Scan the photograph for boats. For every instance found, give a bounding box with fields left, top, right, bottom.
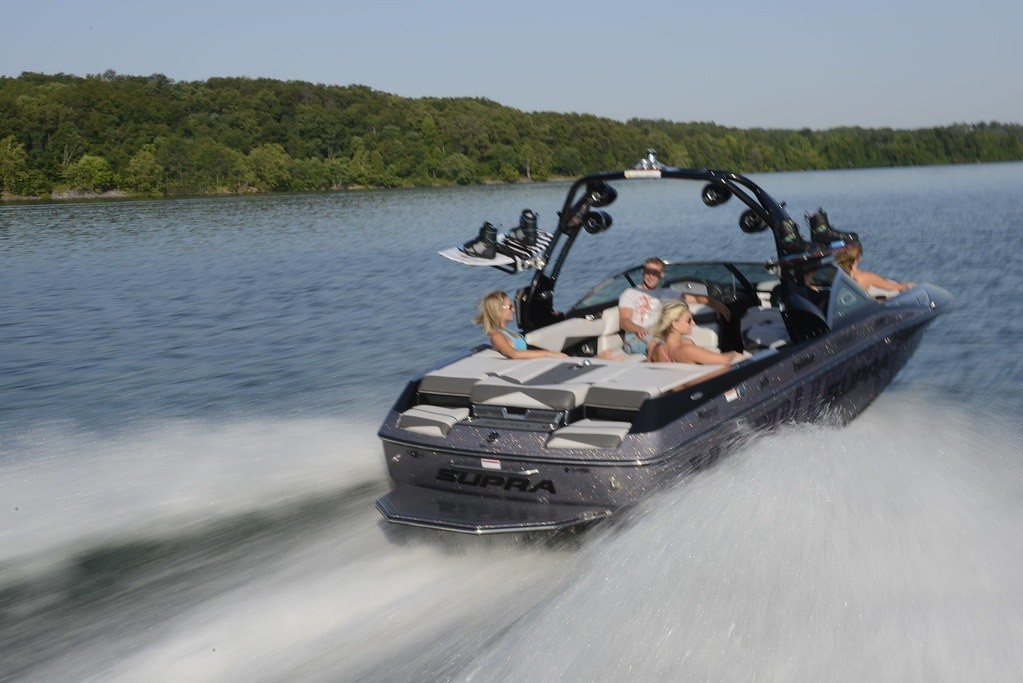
left=373, top=150, right=958, bottom=535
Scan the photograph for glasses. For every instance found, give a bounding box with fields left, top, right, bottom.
left=502, top=304, right=513, bottom=309
left=682, top=318, right=692, bottom=324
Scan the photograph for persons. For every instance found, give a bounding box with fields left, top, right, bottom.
left=470, top=290, right=627, bottom=360
left=839, top=243, right=918, bottom=298
left=646, top=299, right=745, bottom=365
left=618, top=255, right=731, bottom=359
